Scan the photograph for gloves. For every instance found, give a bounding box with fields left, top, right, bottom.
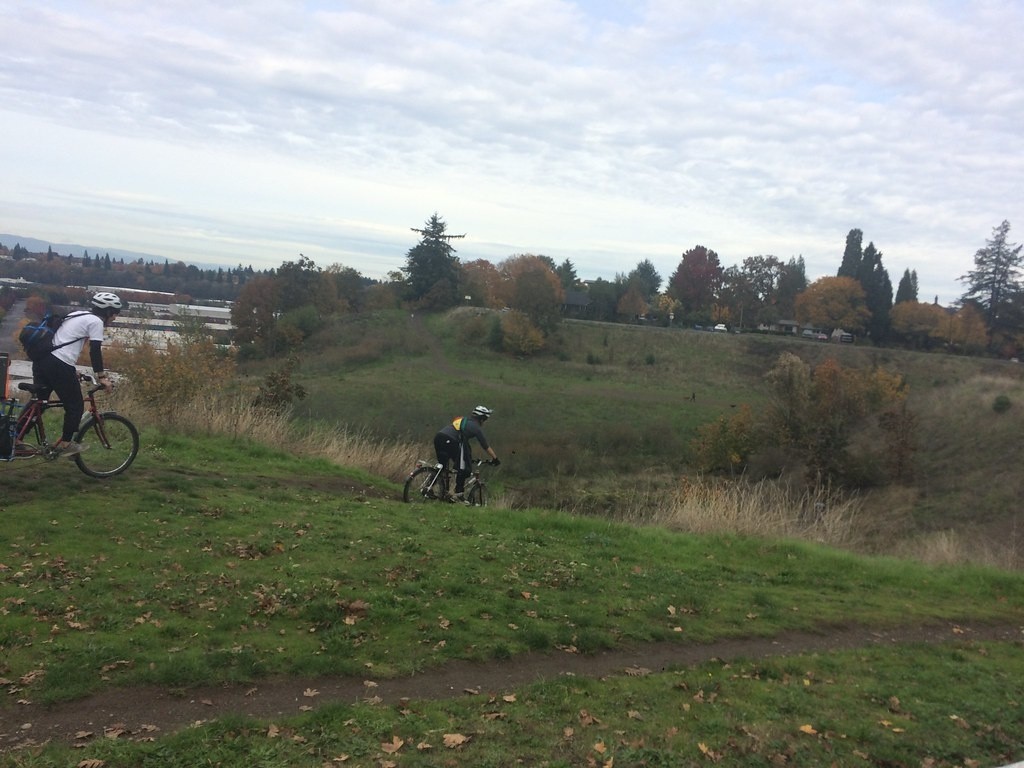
left=490, top=457, right=501, bottom=467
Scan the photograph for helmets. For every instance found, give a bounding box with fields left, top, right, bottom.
left=91, top=292, right=123, bottom=309
left=472, top=405, right=491, bottom=418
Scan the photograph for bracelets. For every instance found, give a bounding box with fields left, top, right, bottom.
left=98, top=375, right=106, bottom=379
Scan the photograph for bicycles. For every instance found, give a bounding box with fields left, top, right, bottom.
left=403, top=455, right=491, bottom=509
left=0, top=373, right=141, bottom=478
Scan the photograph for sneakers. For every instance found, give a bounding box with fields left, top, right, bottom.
left=0, top=446, right=35, bottom=462
left=58, top=440, right=89, bottom=457
left=451, top=494, right=470, bottom=506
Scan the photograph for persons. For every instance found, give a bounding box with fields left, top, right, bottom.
left=434, top=405, right=501, bottom=505
left=12, top=291, right=122, bottom=458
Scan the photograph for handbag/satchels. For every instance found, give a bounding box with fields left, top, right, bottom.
left=456, top=439, right=473, bottom=478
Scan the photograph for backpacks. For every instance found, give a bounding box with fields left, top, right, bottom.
left=19, top=308, right=98, bottom=362
